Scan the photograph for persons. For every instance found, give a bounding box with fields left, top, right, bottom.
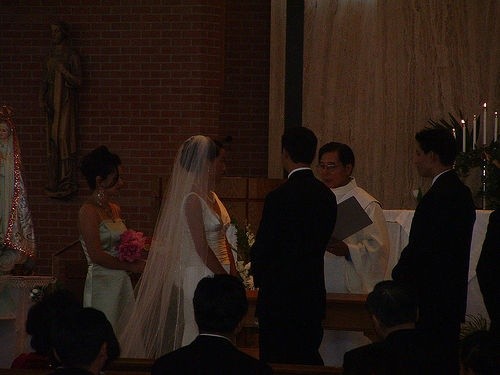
left=149, top=273, right=279, bottom=375
left=242, top=125, right=337, bottom=362
left=341, top=281, right=462, bottom=375
left=78, top=144, right=150, bottom=358
left=476, top=210, right=500, bottom=342
left=112, top=135, right=245, bottom=359
left=392, top=127, right=475, bottom=375
left=11, top=298, right=122, bottom=375
left=0, top=103, right=39, bottom=275
left=41, top=22, right=81, bottom=197
left=318, top=141, right=391, bottom=296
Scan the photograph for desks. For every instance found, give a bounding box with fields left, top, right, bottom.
left=323, top=209, right=496, bottom=339
left=0, top=275, right=57, bottom=370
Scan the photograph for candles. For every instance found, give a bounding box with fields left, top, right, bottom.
left=453, top=128, right=456, bottom=170
left=483, top=103, right=487, bottom=144
left=473, top=114, right=476, bottom=150
left=494, top=112, right=498, bottom=143
left=461, top=120, right=466, bottom=153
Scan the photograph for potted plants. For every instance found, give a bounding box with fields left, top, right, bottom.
left=424, top=108, right=500, bottom=197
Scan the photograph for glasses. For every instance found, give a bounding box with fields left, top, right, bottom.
left=315, top=164, right=342, bottom=171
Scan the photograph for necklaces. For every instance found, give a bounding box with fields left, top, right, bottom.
left=201, top=189, right=218, bottom=204
left=92, top=198, right=119, bottom=223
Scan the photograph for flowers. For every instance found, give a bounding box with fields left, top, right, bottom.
left=118, top=229, right=152, bottom=275
left=412, top=187, right=422, bottom=208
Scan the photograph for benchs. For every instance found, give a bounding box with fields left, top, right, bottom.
left=21, top=357, right=347, bottom=375
